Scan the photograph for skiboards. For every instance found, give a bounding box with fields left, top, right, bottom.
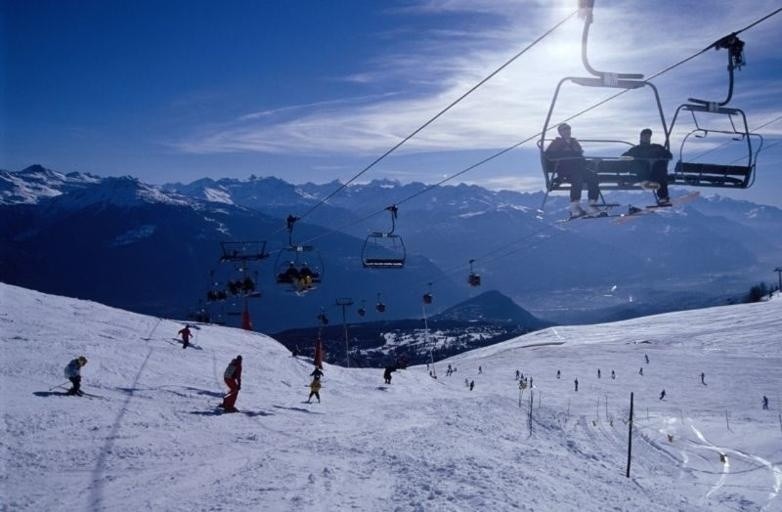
left=213, top=402, right=242, bottom=414
left=613, top=188, right=700, bottom=223
left=559, top=204, right=621, bottom=225
left=59, top=385, right=102, bottom=401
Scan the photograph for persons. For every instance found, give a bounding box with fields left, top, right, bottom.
left=64, top=355, right=88, bottom=397
left=310, top=366, right=323, bottom=381
left=206, top=276, right=255, bottom=303
left=177, top=323, right=194, bottom=350
left=426, top=350, right=706, bottom=402
left=307, top=375, right=322, bottom=403
left=620, top=128, right=674, bottom=205
left=284, top=261, right=300, bottom=287
left=298, top=261, right=315, bottom=285
left=542, top=123, right=609, bottom=218
left=761, top=395, right=770, bottom=411
left=383, top=362, right=396, bottom=384
left=221, top=355, right=243, bottom=413
